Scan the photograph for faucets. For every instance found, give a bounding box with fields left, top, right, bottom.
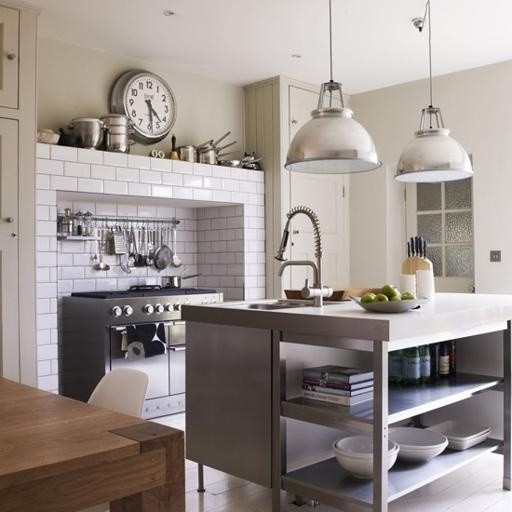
left=278, top=260, right=318, bottom=284
left=273, top=205, right=323, bottom=307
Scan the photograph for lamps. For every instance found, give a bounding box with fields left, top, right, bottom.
left=284, top=0, right=383, bottom=174
left=393, top=0, right=472, bottom=183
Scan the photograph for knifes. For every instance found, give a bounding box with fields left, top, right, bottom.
left=407, top=236, right=427, bottom=260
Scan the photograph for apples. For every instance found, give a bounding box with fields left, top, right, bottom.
left=358, top=283, right=415, bottom=303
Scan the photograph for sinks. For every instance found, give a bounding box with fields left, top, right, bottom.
left=272, top=299, right=332, bottom=307
left=247, top=304, right=286, bottom=310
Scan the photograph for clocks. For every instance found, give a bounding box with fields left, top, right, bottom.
left=108, top=68, right=177, bottom=145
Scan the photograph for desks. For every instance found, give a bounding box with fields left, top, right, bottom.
left=0, top=377, right=185, bottom=512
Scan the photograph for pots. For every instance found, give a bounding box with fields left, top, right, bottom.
left=68, top=118, right=108, bottom=150
left=97, top=113, right=136, bottom=152
left=160, top=272, right=202, bottom=289
left=179, top=130, right=265, bottom=171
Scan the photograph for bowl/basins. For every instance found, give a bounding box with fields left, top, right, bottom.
left=36, top=130, right=60, bottom=145
left=332, top=434, right=400, bottom=480
left=58, top=127, right=82, bottom=147
left=381, top=427, right=448, bottom=463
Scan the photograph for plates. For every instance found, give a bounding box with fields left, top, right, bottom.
left=349, top=295, right=427, bottom=312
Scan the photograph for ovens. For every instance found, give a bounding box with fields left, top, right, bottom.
left=62, top=293, right=225, bottom=420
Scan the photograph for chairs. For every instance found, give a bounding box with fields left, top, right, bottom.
left=87, top=367, right=149, bottom=418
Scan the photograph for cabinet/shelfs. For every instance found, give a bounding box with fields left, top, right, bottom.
left=288, top=84, right=347, bottom=290
left=281, top=371, right=503, bottom=503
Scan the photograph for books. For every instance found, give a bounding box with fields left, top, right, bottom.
left=301, top=365, right=374, bottom=407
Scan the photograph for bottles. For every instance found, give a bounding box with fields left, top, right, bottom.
left=389, top=339, right=456, bottom=386
left=60, top=208, right=94, bottom=235
left=171, top=150, right=179, bottom=161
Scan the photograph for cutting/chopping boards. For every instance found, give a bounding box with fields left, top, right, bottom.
left=284, top=288, right=384, bottom=301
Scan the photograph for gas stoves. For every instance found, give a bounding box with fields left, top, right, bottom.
left=62, top=284, right=223, bottom=324
